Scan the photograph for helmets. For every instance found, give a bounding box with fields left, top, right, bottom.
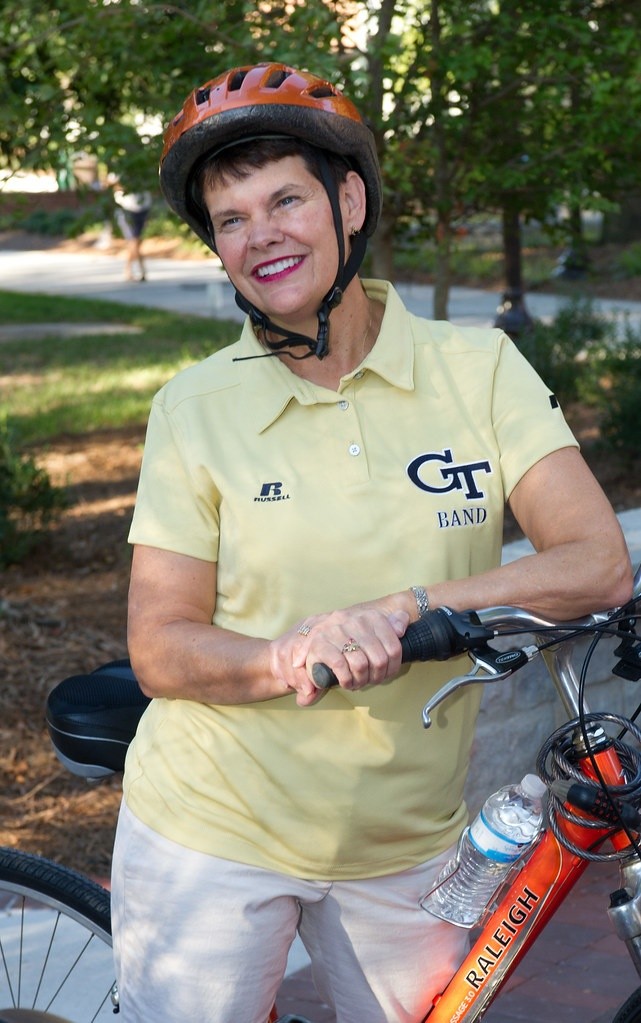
left=159, top=62, right=382, bottom=254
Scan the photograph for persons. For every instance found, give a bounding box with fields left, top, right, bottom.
left=105, top=61, right=639, bottom=1023
left=102, top=164, right=150, bottom=283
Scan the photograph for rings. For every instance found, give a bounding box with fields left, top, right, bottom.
left=297, top=625, right=311, bottom=636
left=342, top=638, right=360, bottom=655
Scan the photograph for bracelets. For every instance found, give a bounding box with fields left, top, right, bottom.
left=409, top=585, right=429, bottom=619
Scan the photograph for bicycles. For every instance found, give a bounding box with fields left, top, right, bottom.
left=2, top=596, right=641, bottom=1023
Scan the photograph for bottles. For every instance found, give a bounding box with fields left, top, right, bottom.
left=431, top=774, right=546, bottom=925
